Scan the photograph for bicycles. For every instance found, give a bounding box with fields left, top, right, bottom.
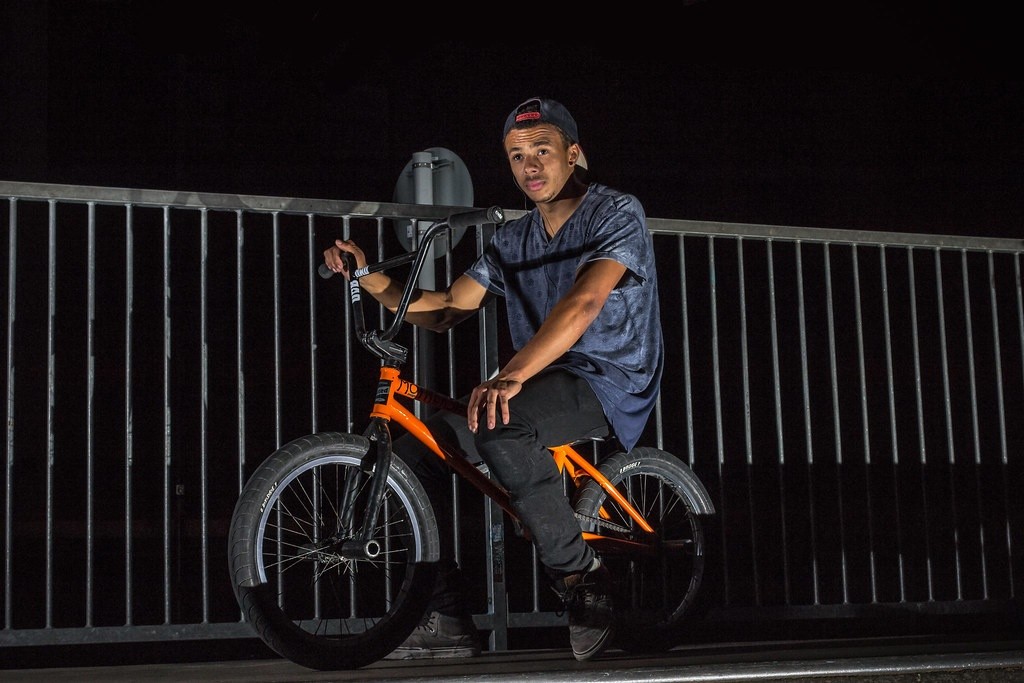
left=226, top=201, right=720, bottom=672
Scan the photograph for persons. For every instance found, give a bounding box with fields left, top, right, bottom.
left=321, top=94, right=669, bottom=667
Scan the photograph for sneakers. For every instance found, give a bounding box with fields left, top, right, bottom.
left=380, top=606, right=482, bottom=658
left=554, top=559, right=622, bottom=661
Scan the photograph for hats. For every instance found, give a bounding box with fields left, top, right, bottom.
left=501, top=97, right=588, bottom=170
